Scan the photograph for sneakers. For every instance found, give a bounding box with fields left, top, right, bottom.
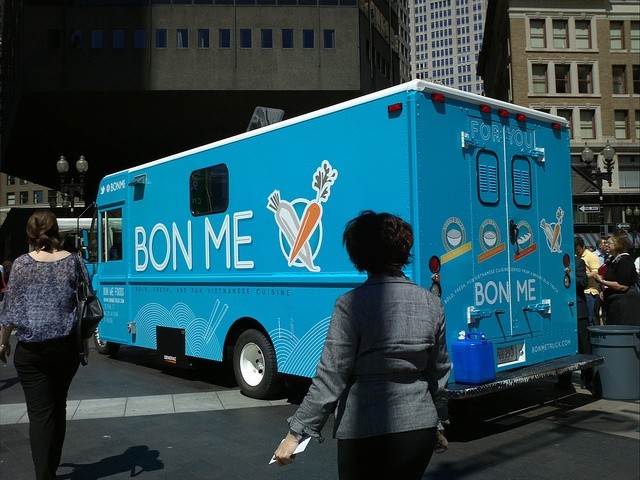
left=580, top=380, right=591, bottom=389
left=553, top=382, right=570, bottom=387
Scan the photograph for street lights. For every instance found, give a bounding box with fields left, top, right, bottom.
left=56, top=154, right=92, bottom=207
left=625, top=204, right=640, bottom=247
left=580, top=140, right=616, bottom=238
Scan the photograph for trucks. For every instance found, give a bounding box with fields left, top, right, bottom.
left=73, top=77, right=605, bottom=431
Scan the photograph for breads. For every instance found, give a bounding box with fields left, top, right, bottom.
left=517, top=233, right=531, bottom=244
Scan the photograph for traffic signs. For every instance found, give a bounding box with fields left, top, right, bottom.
left=578, top=204, right=600, bottom=213
left=616, top=223, right=630, bottom=229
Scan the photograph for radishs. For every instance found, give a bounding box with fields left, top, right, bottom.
left=266, top=189, right=321, bottom=272
left=540, top=219, right=562, bottom=253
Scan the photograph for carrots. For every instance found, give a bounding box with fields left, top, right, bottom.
left=551, top=206, right=564, bottom=250
left=289, top=160, right=337, bottom=264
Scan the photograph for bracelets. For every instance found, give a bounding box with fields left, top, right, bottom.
left=0, top=343, right=12, bottom=348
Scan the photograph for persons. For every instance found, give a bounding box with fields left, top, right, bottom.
left=589, top=245, right=596, bottom=253
left=589, top=239, right=608, bottom=269
left=576, top=236, right=605, bottom=339
left=603, top=235, right=616, bottom=267
left=542, top=239, right=593, bottom=396
left=1, top=259, right=12, bottom=302
left=272, top=209, right=452, bottom=480
left=0, top=207, right=95, bottom=479
left=593, top=233, right=640, bottom=326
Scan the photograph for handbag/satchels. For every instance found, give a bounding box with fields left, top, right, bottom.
left=77, top=280, right=104, bottom=338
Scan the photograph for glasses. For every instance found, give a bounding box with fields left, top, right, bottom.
left=604, top=242, right=617, bottom=246
left=575, top=247, right=581, bottom=253
